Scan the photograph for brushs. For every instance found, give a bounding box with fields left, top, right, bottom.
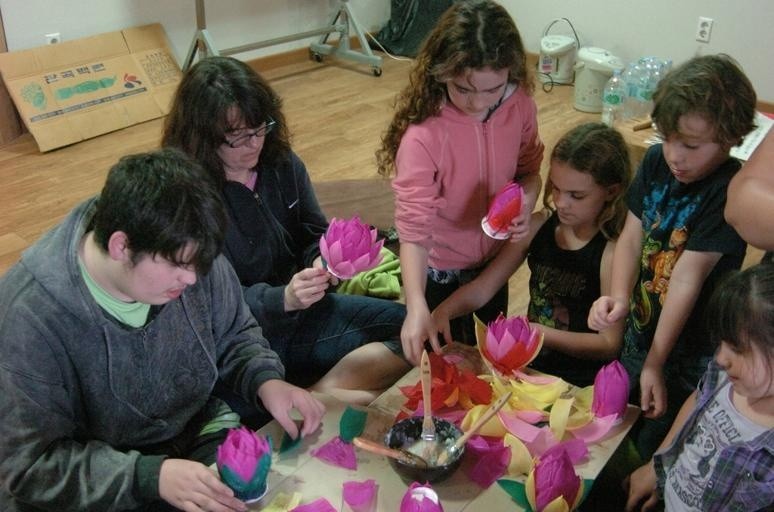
left=421, top=349, right=438, bottom=467
left=353, top=437, right=428, bottom=469
left=436, top=392, right=512, bottom=467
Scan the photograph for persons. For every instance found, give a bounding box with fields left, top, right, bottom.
left=0, top=147, right=328, bottom=512
left=619, top=259, right=773, bottom=512
left=587, top=52, right=757, bottom=463
left=722, top=124, right=774, bottom=264
left=430, top=120, right=633, bottom=389
left=374, top=0, right=545, bottom=365
left=162, top=55, right=414, bottom=405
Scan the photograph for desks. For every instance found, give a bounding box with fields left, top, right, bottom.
left=194, top=338, right=645, bottom=512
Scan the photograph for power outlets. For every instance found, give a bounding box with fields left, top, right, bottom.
left=44, top=33, right=60, bottom=46
left=694, top=17, right=713, bottom=42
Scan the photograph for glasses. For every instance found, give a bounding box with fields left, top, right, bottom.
left=223, top=115, right=276, bottom=147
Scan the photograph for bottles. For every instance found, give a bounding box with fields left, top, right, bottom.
left=601, top=67, right=629, bottom=134
left=625, top=55, right=675, bottom=117
